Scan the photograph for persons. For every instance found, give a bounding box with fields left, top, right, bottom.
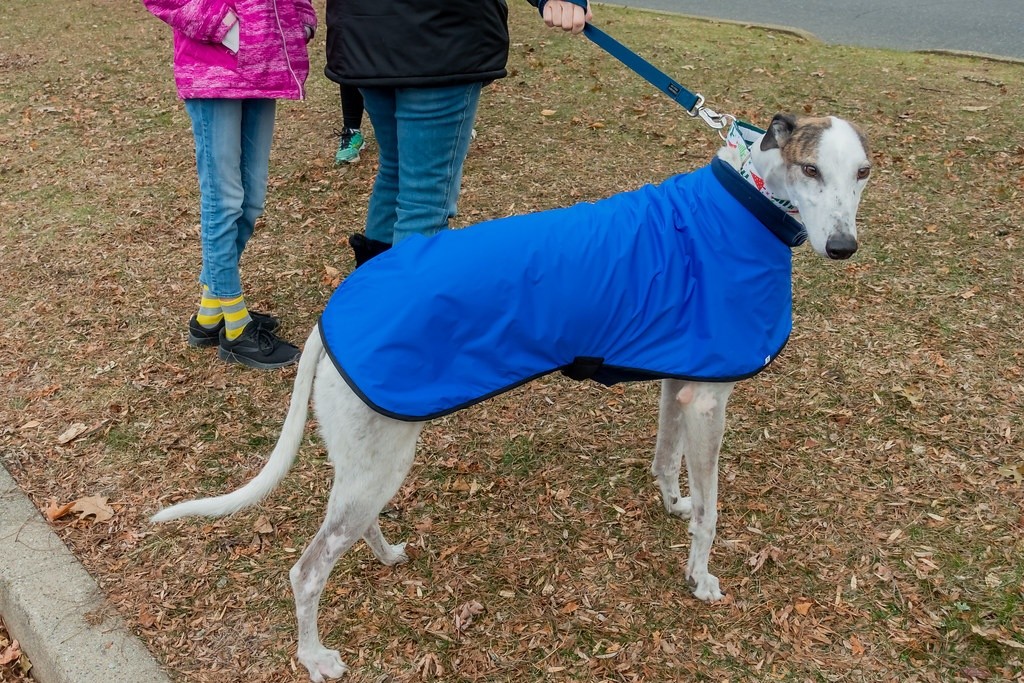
left=336, top=84, right=367, bottom=163
left=142, top=0, right=318, bottom=369
left=324, top=0, right=594, bottom=270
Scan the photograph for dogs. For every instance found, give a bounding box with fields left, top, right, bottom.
left=153, top=115, right=872, bottom=683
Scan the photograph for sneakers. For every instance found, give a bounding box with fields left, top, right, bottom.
left=218, top=321, right=301, bottom=368
left=189, top=311, right=281, bottom=346
left=332, top=127, right=366, bottom=164
